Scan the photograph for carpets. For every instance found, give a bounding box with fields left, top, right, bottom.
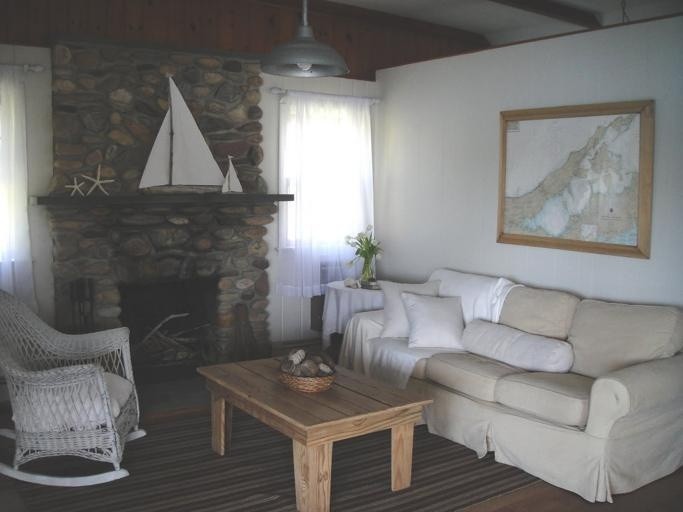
left=0, top=409, right=544, bottom=512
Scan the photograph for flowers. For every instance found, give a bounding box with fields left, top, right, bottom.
left=343, top=224, right=384, bottom=285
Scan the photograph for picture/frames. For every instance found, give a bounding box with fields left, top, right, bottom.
left=495, top=99, right=656, bottom=261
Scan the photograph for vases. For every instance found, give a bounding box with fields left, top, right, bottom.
left=358, top=263, right=376, bottom=285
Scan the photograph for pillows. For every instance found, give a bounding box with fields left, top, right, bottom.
left=401, top=292, right=465, bottom=349
left=567, top=298, right=682, bottom=378
left=498, top=285, right=581, bottom=341
left=378, top=279, right=440, bottom=338
left=460, top=318, right=574, bottom=373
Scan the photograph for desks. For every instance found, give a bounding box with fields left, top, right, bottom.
left=320, top=280, right=386, bottom=352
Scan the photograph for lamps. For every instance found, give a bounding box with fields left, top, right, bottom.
left=260, top=0, right=350, bottom=78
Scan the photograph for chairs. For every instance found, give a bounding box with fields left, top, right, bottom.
left=0, top=289, right=147, bottom=488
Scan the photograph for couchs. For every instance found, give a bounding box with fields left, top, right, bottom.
left=338, top=269, right=682, bottom=505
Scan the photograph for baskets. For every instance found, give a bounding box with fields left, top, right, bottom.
left=281, top=367, right=335, bottom=393
